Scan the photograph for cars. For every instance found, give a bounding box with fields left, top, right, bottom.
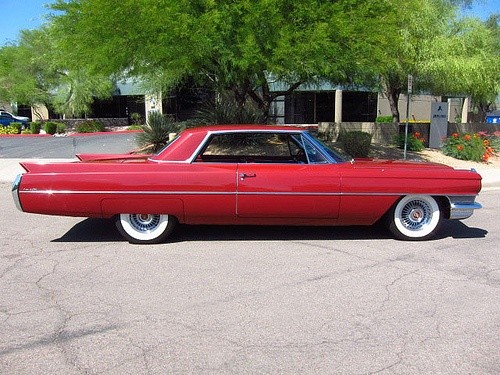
left=0, top=109, right=30, bottom=121
left=10, top=124, right=485, bottom=244
left=0, top=113, right=30, bottom=131
